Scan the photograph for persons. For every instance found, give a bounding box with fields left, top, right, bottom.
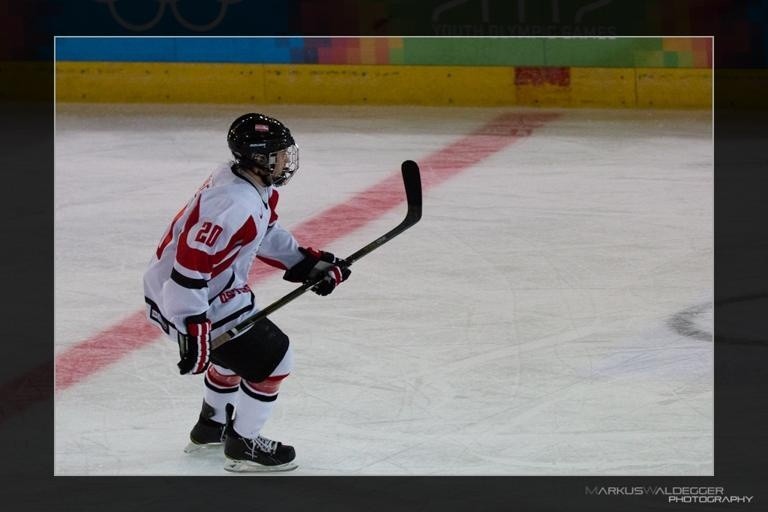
left=145, top=111, right=352, bottom=467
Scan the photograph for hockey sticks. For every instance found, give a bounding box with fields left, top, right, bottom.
left=177, top=159, right=422, bottom=376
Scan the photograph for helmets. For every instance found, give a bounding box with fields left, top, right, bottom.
left=227, top=114, right=300, bottom=187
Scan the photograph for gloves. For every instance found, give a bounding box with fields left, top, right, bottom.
left=177, top=311, right=211, bottom=375
left=283, top=246, right=351, bottom=296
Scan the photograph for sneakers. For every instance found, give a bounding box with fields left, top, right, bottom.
left=190, top=397, right=233, bottom=445
left=224, top=403, right=295, bottom=465
